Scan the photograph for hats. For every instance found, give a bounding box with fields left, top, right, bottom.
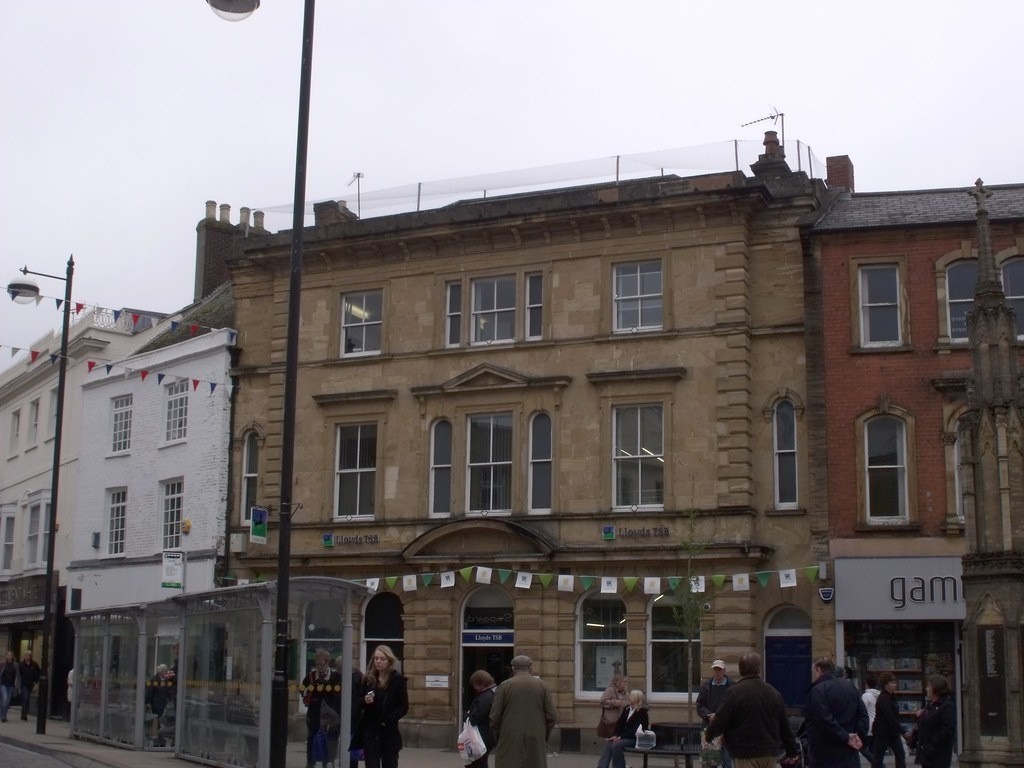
left=511, top=655, right=532, bottom=667
left=469, top=670, right=498, bottom=693
left=711, top=660, right=725, bottom=670
left=927, top=674, right=950, bottom=694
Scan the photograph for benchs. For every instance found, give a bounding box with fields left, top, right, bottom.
left=622, top=716, right=809, bottom=768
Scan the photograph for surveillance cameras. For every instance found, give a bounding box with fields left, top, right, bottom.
left=703, top=603, right=711, bottom=611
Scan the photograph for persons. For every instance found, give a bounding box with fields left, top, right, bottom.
left=298, top=649, right=364, bottom=768
left=0, top=649, right=41, bottom=722
left=465, top=671, right=498, bottom=768
left=145, top=663, right=169, bottom=746
left=596, top=674, right=649, bottom=768
left=697, top=660, right=736, bottom=768
left=804, top=656, right=870, bottom=768
left=705, top=652, right=799, bottom=768
left=165, top=641, right=198, bottom=718
left=914, top=675, right=957, bottom=768
left=347, top=645, right=410, bottom=768
left=489, top=655, right=557, bottom=768
left=861, top=673, right=911, bottom=768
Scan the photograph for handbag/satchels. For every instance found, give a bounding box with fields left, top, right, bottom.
left=597, top=715, right=619, bottom=739
left=905, top=725, right=918, bottom=748
left=303, top=688, right=313, bottom=707
left=350, top=735, right=366, bottom=762
left=779, top=754, right=801, bottom=768
left=634, top=724, right=656, bottom=749
left=457, top=717, right=487, bottom=766
left=312, top=726, right=330, bottom=762
left=698, top=729, right=724, bottom=763
left=145, top=707, right=159, bottom=741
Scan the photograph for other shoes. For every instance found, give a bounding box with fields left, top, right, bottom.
left=1, top=719, right=7, bottom=723
left=21, top=716, right=27, bottom=721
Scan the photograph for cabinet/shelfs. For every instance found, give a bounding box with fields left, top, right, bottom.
left=867, top=665, right=923, bottom=716
